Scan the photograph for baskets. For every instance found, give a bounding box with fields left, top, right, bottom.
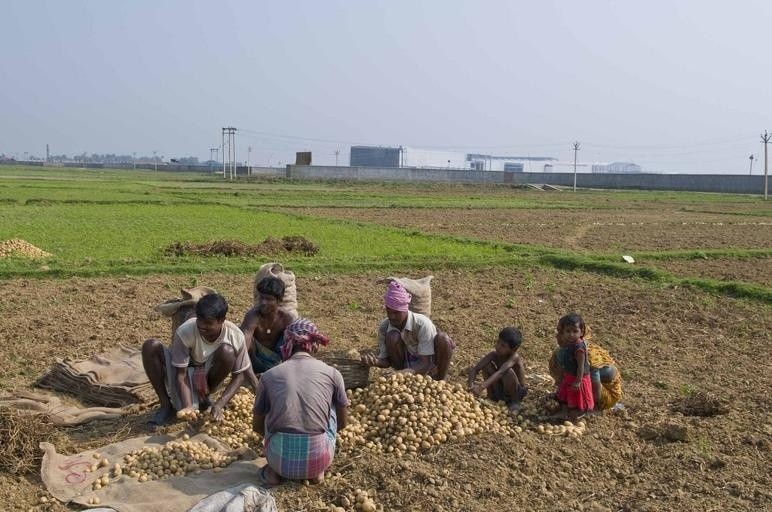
left=321, top=357, right=368, bottom=389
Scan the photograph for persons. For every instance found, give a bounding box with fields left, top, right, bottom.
left=557, top=313, right=595, bottom=412
left=252, top=318, right=352, bottom=485
left=361, top=281, right=456, bottom=380
left=241, top=276, right=296, bottom=392
left=549, top=323, right=622, bottom=411
left=467, top=327, right=529, bottom=413
left=141, top=294, right=252, bottom=431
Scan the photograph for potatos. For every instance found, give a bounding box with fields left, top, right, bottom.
left=39, top=372, right=586, bottom=512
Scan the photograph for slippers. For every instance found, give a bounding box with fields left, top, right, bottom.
left=200, top=399, right=227, bottom=410
left=258, top=464, right=280, bottom=487
left=146, top=407, right=177, bottom=431
